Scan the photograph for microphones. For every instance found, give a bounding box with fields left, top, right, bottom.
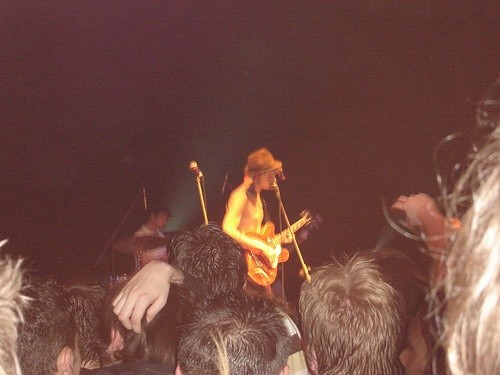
left=190, top=160, right=203, bottom=177
left=143, top=188, right=147, bottom=209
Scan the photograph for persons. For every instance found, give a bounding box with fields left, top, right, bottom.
left=0, top=146, right=500, bottom=375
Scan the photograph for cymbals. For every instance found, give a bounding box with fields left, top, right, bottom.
left=111, top=236, right=166, bottom=251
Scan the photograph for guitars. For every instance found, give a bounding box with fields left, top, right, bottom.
left=240, top=209, right=314, bottom=287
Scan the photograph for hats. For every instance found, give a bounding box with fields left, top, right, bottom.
left=244, top=149, right=282, bottom=176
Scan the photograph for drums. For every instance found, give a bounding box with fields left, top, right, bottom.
left=107, top=270, right=135, bottom=290
